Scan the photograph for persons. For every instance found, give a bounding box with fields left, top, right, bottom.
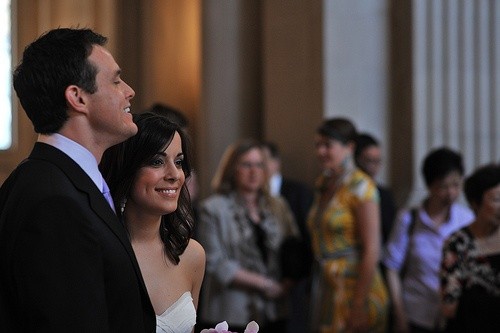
left=1, top=24, right=157, bottom=333
left=349, top=132, right=381, bottom=177
left=192, top=138, right=301, bottom=333
left=138, top=102, right=200, bottom=202
left=304, top=118, right=391, bottom=332
left=439, top=161, right=500, bottom=332
left=254, top=141, right=313, bottom=333
left=384, top=145, right=476, bottom=333
left=97, top=112, right=206, bottom=332
